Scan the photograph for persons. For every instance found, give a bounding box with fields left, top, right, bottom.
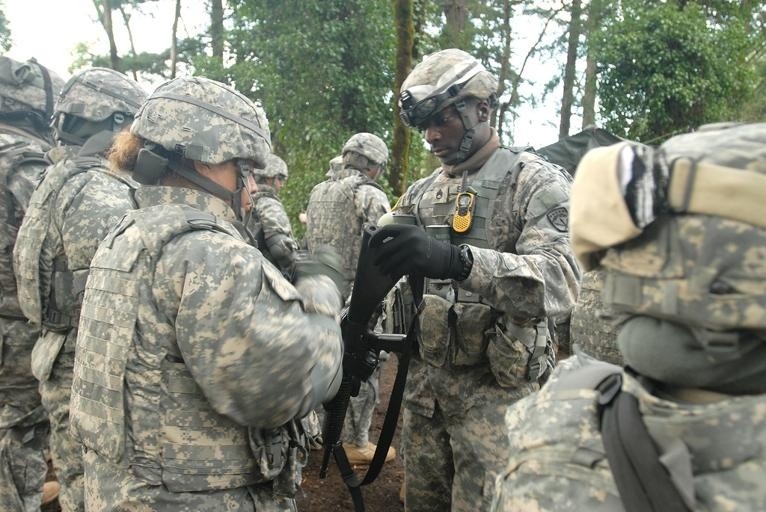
left=480, top=123, right=766, bottom=512
left=69, top=74, right=343, bottom=512
left=13, top=65, right=149, bottom=512
left=316, top=46, right=586, bottom=509
left=299, top=153, right=342, bottom=225
left=1, top=55, right=66, bottom=512
left=250, top=154, right=301, bottom=285
left=304, top=131, right=395, bottom=468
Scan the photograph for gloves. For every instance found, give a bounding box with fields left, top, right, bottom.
left=369, top=224, right=453, bottom=282
left=287, top=246, right=350, bottom=290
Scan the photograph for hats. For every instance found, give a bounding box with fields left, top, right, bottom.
left=324, top=157, right=342, bottom=179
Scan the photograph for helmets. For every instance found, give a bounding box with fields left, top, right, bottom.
left=342, top=132, right=391, bottom=167
left=0, top=55, right=58, bottom=136
left=598, top=122, right=766, bottom=394
left=398, top=48, right=499, bottom=126
left=50, top=68, right=146, bottom=143
left=132, top=75, right=272, bottom=170
left=253, top=155, right=288, bottom=184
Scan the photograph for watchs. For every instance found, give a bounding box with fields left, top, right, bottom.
left=458, top=245, right=473, bottom=282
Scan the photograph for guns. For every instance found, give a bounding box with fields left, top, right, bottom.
left=318, top=222, right=408, bottom=481
